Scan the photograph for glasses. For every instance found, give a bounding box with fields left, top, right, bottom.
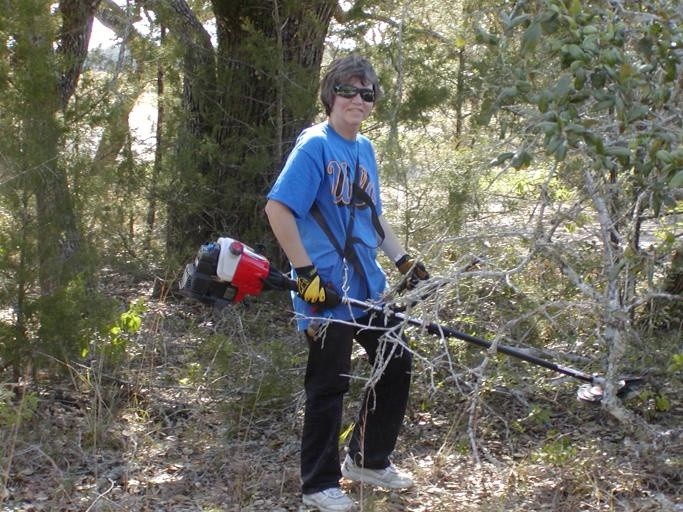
left=334, top=84, right=374, bottom=102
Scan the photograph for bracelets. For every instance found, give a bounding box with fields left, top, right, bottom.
left=393, top=252, right=410, bottom=263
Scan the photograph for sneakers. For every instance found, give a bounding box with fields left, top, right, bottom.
left=302, top=488, right=358, bottom=512
left=341, top=454, right=414, bottom=490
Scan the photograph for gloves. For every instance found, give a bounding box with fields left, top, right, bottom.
left=295, top=265, right=326, bottom=304
left=394, top=254, right=429, bottom=290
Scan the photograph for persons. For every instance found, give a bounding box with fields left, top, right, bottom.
left=264, top=55, right=430, bottom=512
